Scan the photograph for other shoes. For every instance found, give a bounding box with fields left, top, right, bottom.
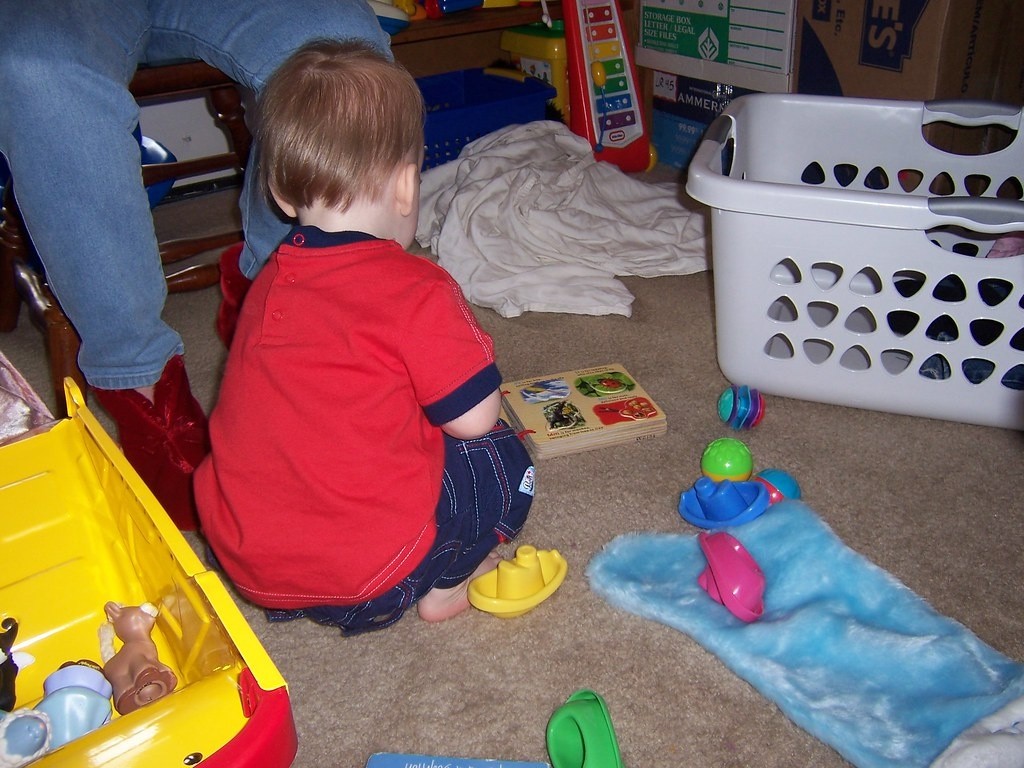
left=92, top=355, right=211, bottom=533
left=216, top=243, right=257, bottom=348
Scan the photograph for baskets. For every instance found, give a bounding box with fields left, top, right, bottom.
left=413, top=65, right=557, bottom=172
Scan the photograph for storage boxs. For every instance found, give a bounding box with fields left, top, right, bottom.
left=414, top=65, right=559, bottom=174
left=644, top=69, right=761, bottom=172
left=635, top=0, right=799, bottom=91
left=684, top=94, right=1024, bottom=429
left=791, top=0, right=1024, bottom=205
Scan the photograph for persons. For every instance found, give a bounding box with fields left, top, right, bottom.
left=0, top=0, right=395, bottom=525
left=193, top=35, right=536, bottom=636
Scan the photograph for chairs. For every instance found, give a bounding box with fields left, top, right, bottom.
left=0, top=58, right=256, bottom=423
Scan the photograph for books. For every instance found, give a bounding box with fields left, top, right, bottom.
left=499, top=362, right=668, bottom=458
